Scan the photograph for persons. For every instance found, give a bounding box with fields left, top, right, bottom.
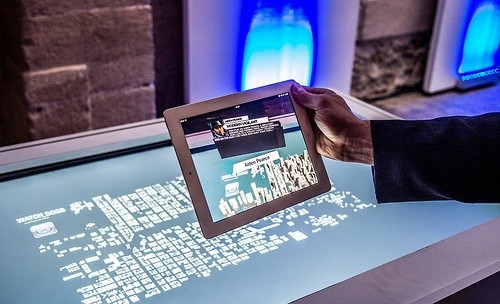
left=210, top=120, right=230, bottom=138
left=291, top=84, right=500, bottom=205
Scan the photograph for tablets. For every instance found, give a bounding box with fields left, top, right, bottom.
left=163, top=79, right=332, bottom=239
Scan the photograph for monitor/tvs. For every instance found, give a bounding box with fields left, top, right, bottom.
left=0, top=87, right=500, bottom=304
